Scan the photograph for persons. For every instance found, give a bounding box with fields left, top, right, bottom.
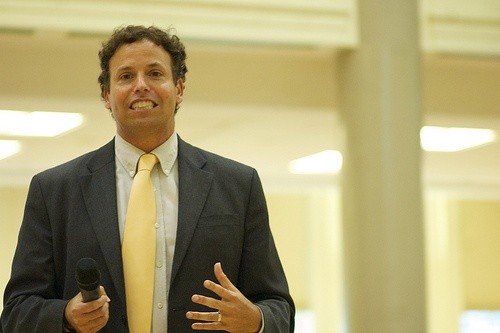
left=0, top=22, right=297, bottom=333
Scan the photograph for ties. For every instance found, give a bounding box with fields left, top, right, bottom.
left=122, top=154, right=160, bottom=332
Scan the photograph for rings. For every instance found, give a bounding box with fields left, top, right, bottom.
left=217, top=311, right=222, bottom=322
